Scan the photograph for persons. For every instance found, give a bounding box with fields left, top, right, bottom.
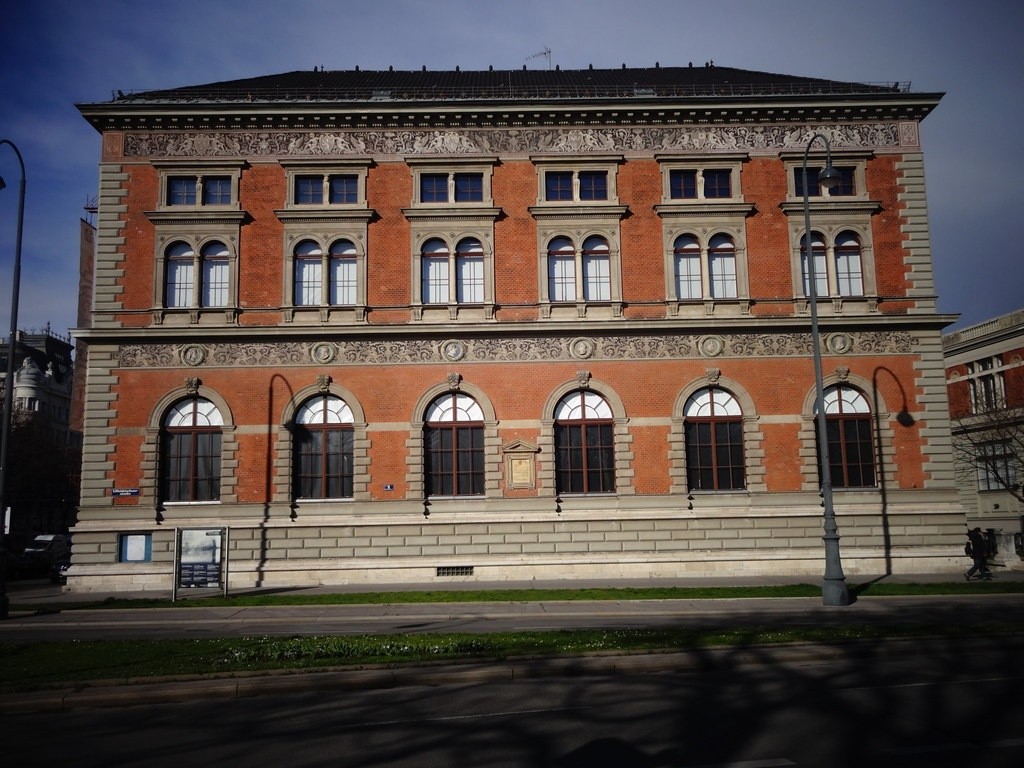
left=575, top=341, right=590, bottom=355
left=705, top=339, right=719, bottom=353
left=318, top=347, right=330, bottom=361
left=446, top=344, right=457, bottom=357
left=833, top=336, right=847, bottom=350
left=186, top=348, right=197, bottom=364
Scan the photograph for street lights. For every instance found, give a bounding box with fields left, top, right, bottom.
left=803, top=133, right=851, bottom=607
left=0, top=138, right=25, bottom=620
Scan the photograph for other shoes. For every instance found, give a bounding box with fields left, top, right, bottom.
left=979, top=576, right=984, bottom=580
left=963, top=572, right=972, bottom=582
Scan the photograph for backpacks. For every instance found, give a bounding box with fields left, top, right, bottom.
left=964, top=542, right=974, bottom=557
